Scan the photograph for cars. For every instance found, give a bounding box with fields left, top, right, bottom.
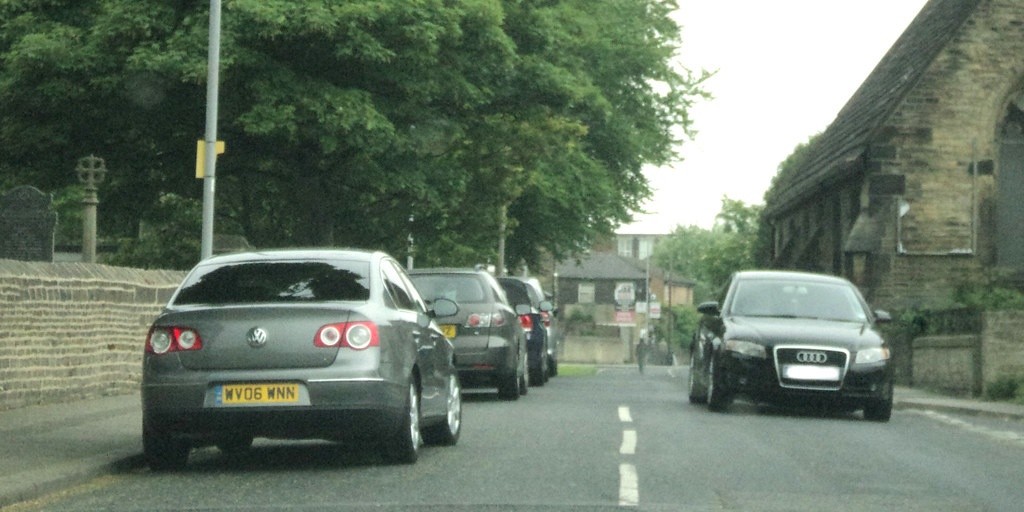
left=138, top=249, right=463, bottom=464
left=497, top=276, right=560, bottom=388
left=686, top=270, right=895, bottom=422
left=405, top=264, right=534, bottom=400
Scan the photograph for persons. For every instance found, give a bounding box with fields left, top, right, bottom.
left=637, top=338, right=647, bottom=373
